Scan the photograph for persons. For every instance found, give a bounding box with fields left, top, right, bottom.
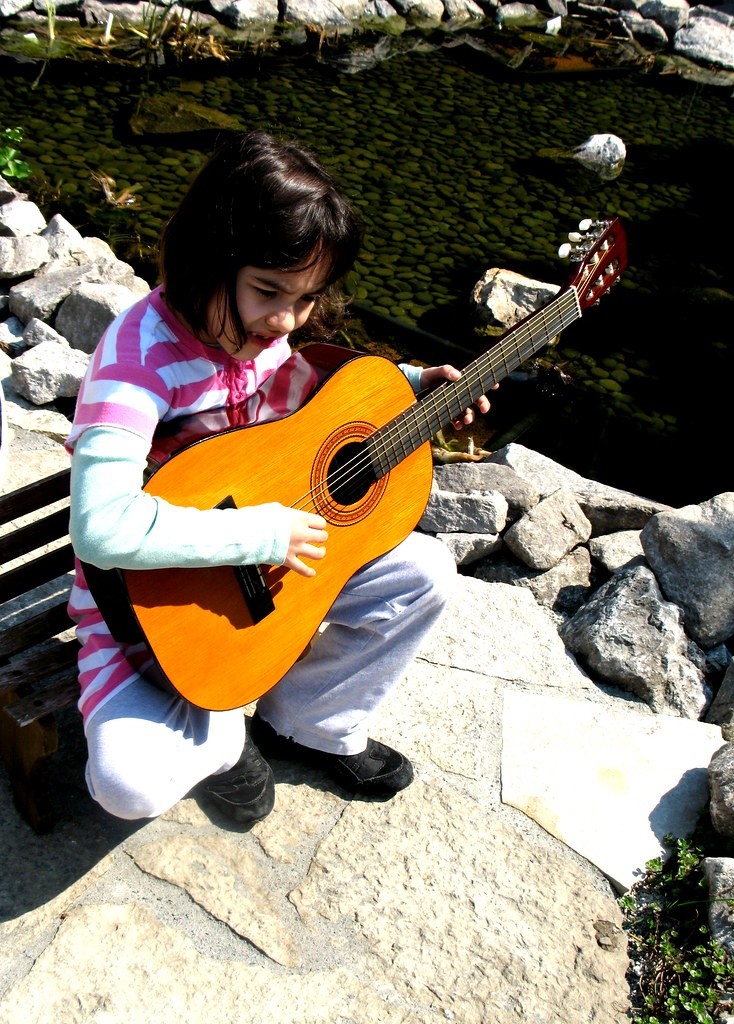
left=68, top=135, right=501, bottom=825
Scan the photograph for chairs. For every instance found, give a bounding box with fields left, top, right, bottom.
left=0, top=468, right=87, bottom=833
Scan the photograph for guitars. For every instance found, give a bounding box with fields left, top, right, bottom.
left=82, top=217, right=627, bottom=710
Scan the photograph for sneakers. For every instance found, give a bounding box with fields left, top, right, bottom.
left=250, top=710, right=414, bottom=797
left=199, top=721, right=275, bottom=823
